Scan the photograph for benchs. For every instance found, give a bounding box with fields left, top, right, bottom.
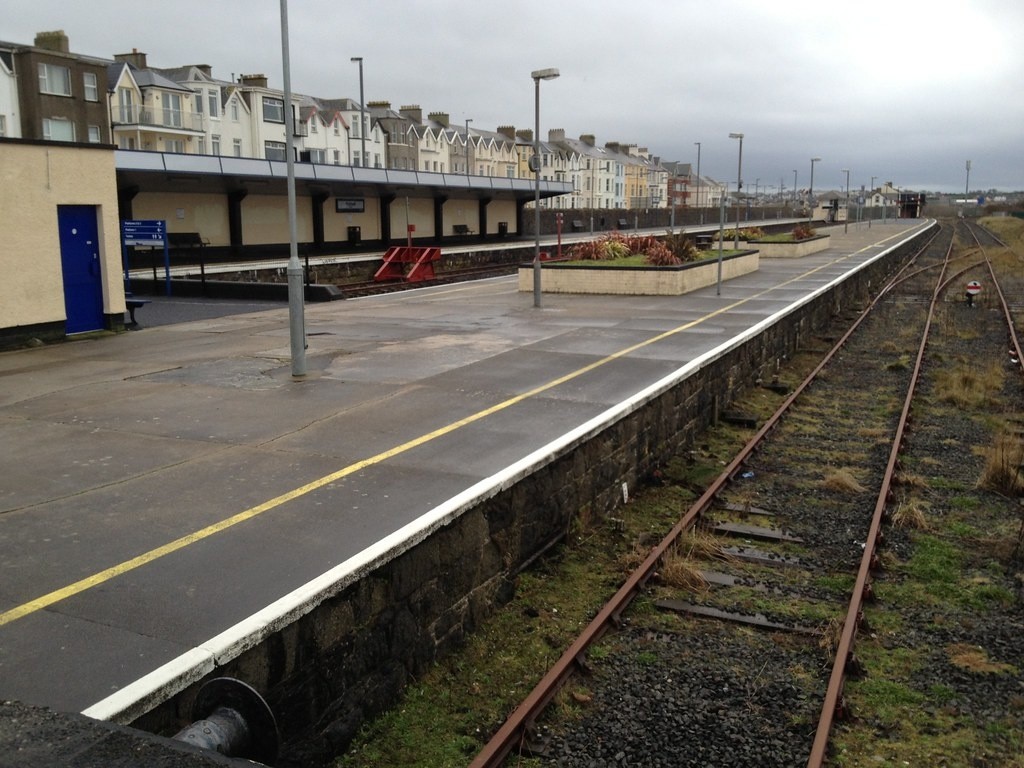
left=618, top=219, right=628, bottom=229
left=165, top=233, right=211, bottom=259
left=572, top=221, right=586, bottom=232
left=452, top=225, right=474, bottom=239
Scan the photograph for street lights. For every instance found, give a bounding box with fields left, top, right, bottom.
left=792, top=169, right=798, bottom=211
left=350, top=57, right=368, bottom=167
left=694, top=141, right=701, bottom=210
left=869, top=176, right=878, bottom=227
left=841, top=169, right=851, bottom=234
left=578, top=158, right=607, bottom=236
left=895, top=186, right=903, bottom=220
left=529, top=68, right=560, bottom=306
left=755, top=178, right=761, bottom=209
left=883, top=183, right=888, bottom=223
left=729, top=133, right=744, bottom=250
left=623, top=162, right=651, bottom=235
left=465, top=119, right=474, bottom=176
left=808, top=157, right=823, bottom=235
